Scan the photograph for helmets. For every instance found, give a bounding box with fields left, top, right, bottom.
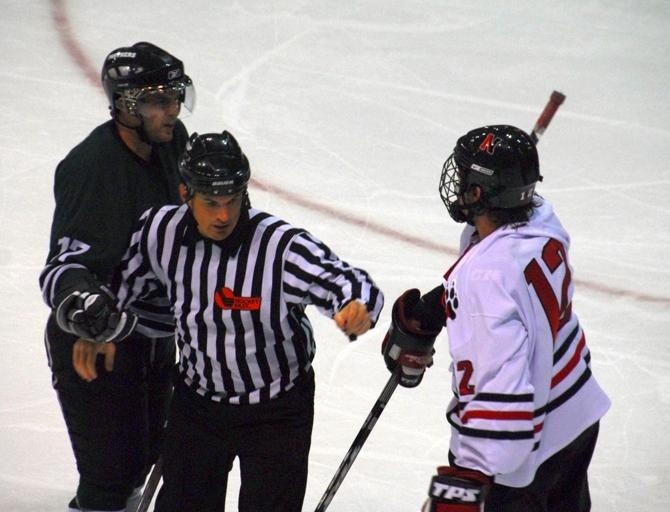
left=438, top=125, right=543, bottom=226
left=101, top=42, right=195, bottom=117
left=175, top=130, right=251, bottom=198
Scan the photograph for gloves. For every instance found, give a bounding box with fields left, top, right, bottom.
left=381, top=288, right=444, bottom=388
left=422, top=466, right=495, bottom=512
left=51, top=278, right=140, bottom=344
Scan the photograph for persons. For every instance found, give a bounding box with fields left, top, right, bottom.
left=379, top=122, right=610, bottom=512
left=71, top=130, right=388, bottom=512
left=35, top=41, right=191, bottom=512
